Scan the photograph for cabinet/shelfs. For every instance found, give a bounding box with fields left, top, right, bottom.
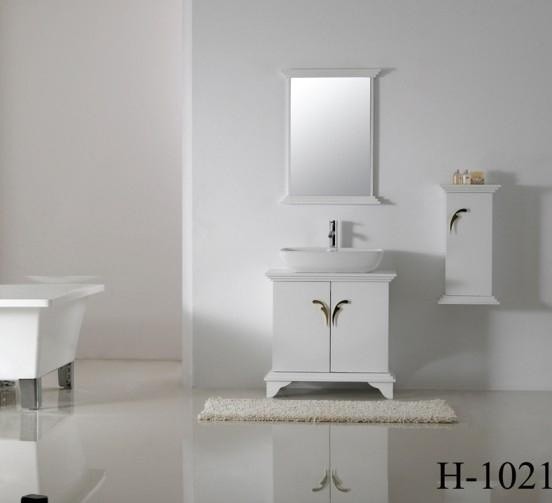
left=274, top=424, right=389, bottom=503
left=437, top=184, right=502, bottom=304
left=272, top=282, right=389, bottom=373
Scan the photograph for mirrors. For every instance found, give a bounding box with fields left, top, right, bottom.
left=280, top=67, right=383, bottom=205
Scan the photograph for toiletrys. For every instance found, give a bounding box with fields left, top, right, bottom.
left=453, top=168, right=463, bottom=184
left=462, top=169, right=472, bottom=185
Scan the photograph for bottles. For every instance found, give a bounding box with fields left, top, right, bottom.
left=453, top=169, right=472, bottom=184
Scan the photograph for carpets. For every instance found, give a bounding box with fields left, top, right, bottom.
left=198, top=395, right=457, bottom=423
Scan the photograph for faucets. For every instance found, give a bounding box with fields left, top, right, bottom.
left=327, top=218, right=336, bottom=247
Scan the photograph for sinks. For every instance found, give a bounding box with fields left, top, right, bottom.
left=280, top=247, right=382, bottom=272
